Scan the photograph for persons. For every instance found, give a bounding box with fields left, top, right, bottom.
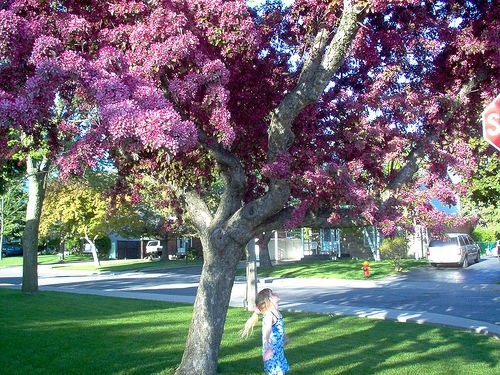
left=255, top=288, right=290, bottom=375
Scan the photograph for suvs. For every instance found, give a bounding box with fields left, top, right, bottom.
left=145, top=240, right=164, bottom=254
left=426, top=233, right=481, bottom=268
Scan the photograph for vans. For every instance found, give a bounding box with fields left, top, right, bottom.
left=1, top=245, right=23, bottom=257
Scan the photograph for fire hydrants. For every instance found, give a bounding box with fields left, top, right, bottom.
left=362, top=260, right=371, bottom=277
